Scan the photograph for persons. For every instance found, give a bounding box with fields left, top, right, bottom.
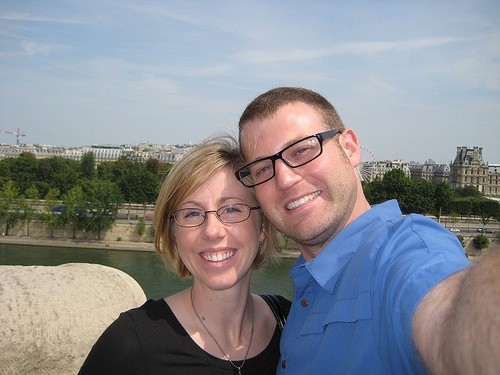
left=238, top=87, right=500, bottom=375
left=75, top=135, right=292, bottom=375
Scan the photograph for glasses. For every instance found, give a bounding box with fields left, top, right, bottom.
left=168, top=205, right=259, bottom=227
left=234, top=127, right=343, bottom=188
